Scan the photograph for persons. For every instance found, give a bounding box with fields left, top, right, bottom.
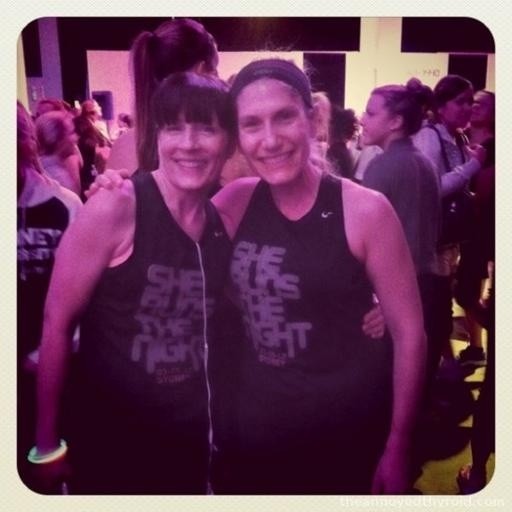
left=16, top=18, right=494, bottom=496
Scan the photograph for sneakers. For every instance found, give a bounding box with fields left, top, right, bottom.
left=453, top=345, right=488, bottom=369
